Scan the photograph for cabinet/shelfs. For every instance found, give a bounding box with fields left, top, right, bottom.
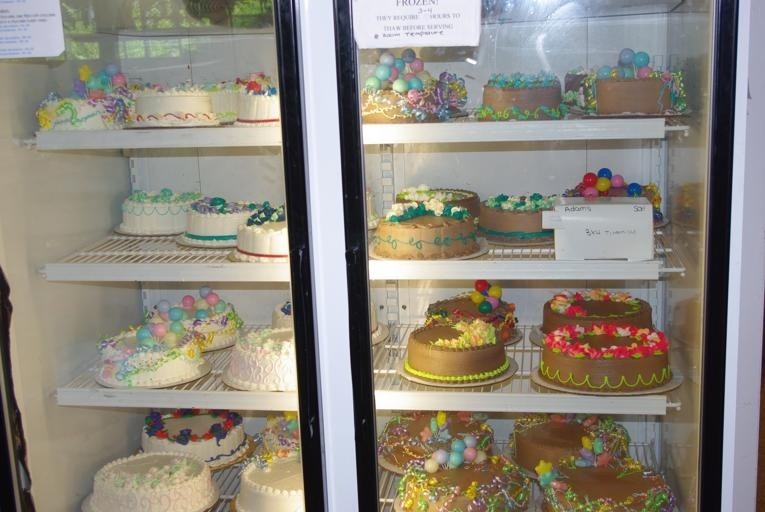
left=0, top=0, right=725, bottom=512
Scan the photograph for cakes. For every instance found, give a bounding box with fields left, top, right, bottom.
left=377, top=411, right=679, bottom=512
left=359, top=48, right=673, bottom=125
left=90, top=408, right=306, bottom=512
left=365, top=168, right=661, bottom=261
left=96, top=285, right=298, bottom=393
left=36, top=62, right=282, bottom=132
left=370, top=280, right=673, bottom=392
left=119, top=184, right=291, bottom=262
